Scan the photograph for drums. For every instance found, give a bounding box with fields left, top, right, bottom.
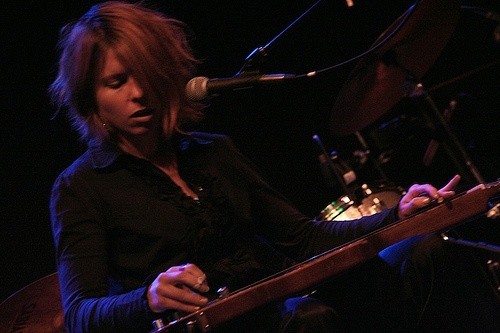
left=310, top=182, right=406, bottom=222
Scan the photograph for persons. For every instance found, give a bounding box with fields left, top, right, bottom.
left=48, top=0, right=460, bottom=333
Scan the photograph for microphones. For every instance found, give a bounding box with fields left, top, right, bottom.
left=182, top=72, right=294, bottom=102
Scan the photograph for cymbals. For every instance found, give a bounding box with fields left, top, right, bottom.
left=1, top=271, right=64, bottom=333
left=331, top=62, right=407, bottom=140
